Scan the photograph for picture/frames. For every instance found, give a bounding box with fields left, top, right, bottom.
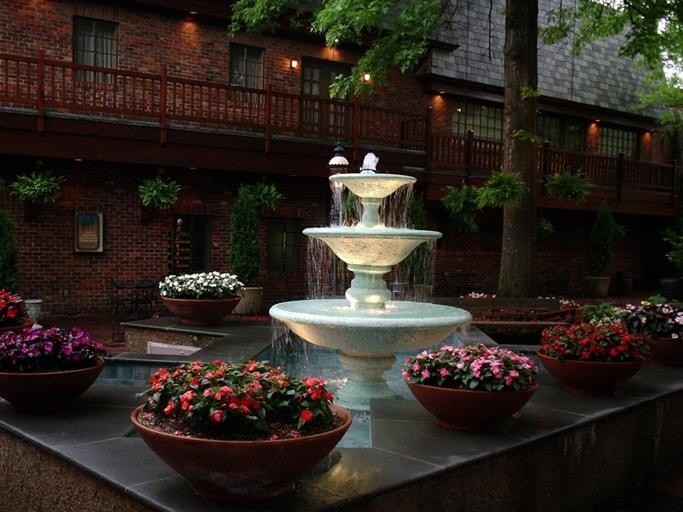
left=72, top=207, right=104, bottom=254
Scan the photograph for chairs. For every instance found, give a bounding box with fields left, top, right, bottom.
left=102, top=270, right=158, bottom=343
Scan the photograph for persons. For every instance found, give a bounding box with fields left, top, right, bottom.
left=613, top=271, right=626, bottom=306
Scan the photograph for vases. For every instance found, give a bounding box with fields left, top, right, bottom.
left=2, top=359, right=106, bottom=408
left=159, top=295, right=243, bottom=329
left=406, top=375, right=540, bottom=434
left=530, top=346, right=633, bottom=396
left=4, top=326, right=31, bottom=334
left=130, top=400, right=354, bottom=497
left=24, top=299, right=43, bottom=324
left=644, top=333, right=682, bottom=367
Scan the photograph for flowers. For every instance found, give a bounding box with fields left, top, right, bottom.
left=0, top=289, right=32, bottom=321
left=535, top=321, right=641, bottom=363
left=0, top=324, right=106, bottom=367
left=133, top=355, right=339, bottom=442
left=616, top=296, right=682, bottom=337
left=401, top=340, right=539, bottom=392
left=157, top=269, right=246, bottom=299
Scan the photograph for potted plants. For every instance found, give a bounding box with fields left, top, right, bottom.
left=217, top=179, right=283, bottom=315
left=576, top=198, right=630, bottom=297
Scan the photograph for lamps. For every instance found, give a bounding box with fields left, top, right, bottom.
left=288, top=54, right=298, bottom=72
